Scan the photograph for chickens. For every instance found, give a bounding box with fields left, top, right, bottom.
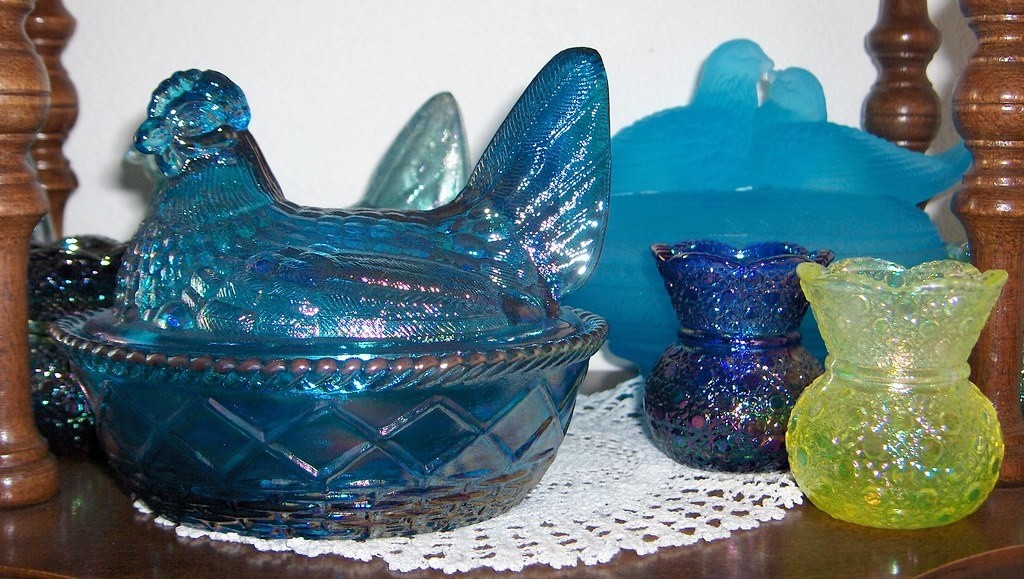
left=115, top=46, right=612, bottom=340
